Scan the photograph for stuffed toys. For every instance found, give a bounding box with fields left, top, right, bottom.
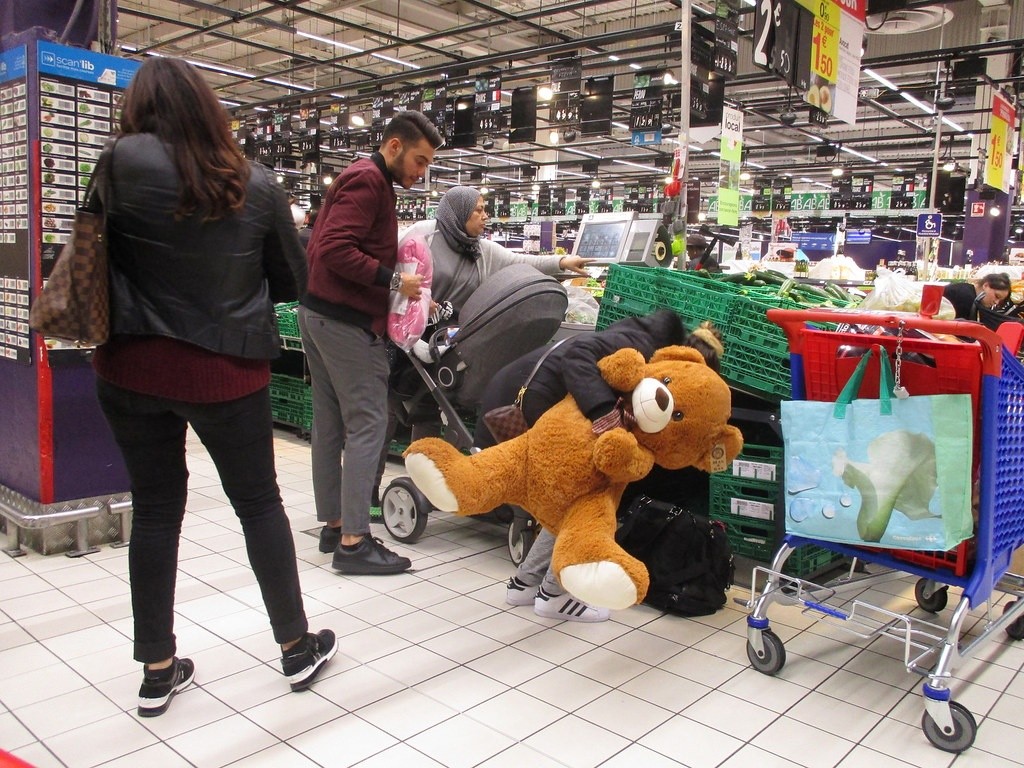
left=401, top=346, right=745, bottom=611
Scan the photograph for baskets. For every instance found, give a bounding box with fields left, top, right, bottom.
left=263, top=298, right=477, bottom=457
left=708, top=442, right=844, bottom=577
left=595, top=262, right=865, bottom=400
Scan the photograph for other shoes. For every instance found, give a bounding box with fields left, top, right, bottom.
left=368, top=505, right=386, bottom=524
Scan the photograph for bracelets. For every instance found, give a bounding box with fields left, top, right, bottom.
left=389, top=272, right=402, bottom=290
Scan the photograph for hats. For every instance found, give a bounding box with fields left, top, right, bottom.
left=686, top=234, right=710, bottom=248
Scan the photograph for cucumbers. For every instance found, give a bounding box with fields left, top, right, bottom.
left=699, top=268, right=856, bottom=308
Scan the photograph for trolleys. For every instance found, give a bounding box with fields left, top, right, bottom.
left=743, top=309, right=1024, bottom=754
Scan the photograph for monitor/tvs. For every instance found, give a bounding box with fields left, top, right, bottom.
left=570, top=210, right=639, bottom=265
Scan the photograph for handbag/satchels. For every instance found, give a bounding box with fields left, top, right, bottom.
left=482, top=404, right=529, bottom=444
left=780, top=345, right=974, bottom=553
left=614, top=494, right=735, bottom=616
left=27, top=131, right=125, bottom=347
left=859, top=264, right=956, bottom=321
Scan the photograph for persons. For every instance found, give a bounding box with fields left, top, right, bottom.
left=471, top=311, right=726, bottom=622
left=286, top=193, right=305, bottom=226
left=942, top=272, right=1010, bottom=320
left=673, top=235, right=720, bottom=272
left=299, top=109, right=444, bottom=573
left=371, top=184, right=597, bottom=525
left=89, top=57, right=339, bottom=718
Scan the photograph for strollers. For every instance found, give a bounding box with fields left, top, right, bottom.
left=379, top=261, right=570, bottom=569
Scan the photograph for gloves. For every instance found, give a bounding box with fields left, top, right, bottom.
left=591, top=403, right=625, bottom=440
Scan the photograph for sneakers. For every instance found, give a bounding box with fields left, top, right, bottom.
left=138, top=655, right=195, bottom=717
left=280, top=628, right=338, bottom=692
left=506, top=577, right=540, bottom=606
left=332, top=533, right=411, bottom=574
left=535, top=585, right=610, bottom=622
left=319, top=524, right=342, bottom=553
